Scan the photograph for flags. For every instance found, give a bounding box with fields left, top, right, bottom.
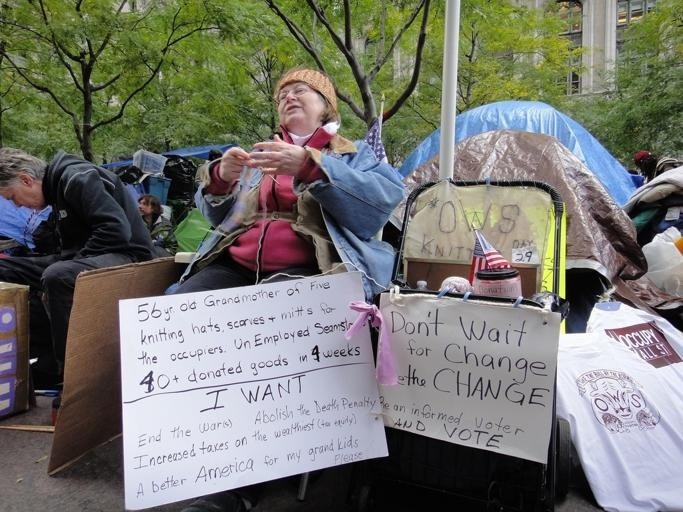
left=363, top=108, right=389, bottom=164
left=468, top=228, right=512, bottom=287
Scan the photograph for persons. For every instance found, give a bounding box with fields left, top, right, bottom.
left=162, top=65, right=406, bottom=512
left=137, top=194, right=172, bottom=246
left=630, top=150, right=678, bottom=185
left=193, top=148, right=223, bottom=189
left=0, top=144, right=155, bottom=409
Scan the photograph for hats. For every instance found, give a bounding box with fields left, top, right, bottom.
left=634, top=150, right=652, bottom=164
left=274, top=68, right=338, bottom=110
